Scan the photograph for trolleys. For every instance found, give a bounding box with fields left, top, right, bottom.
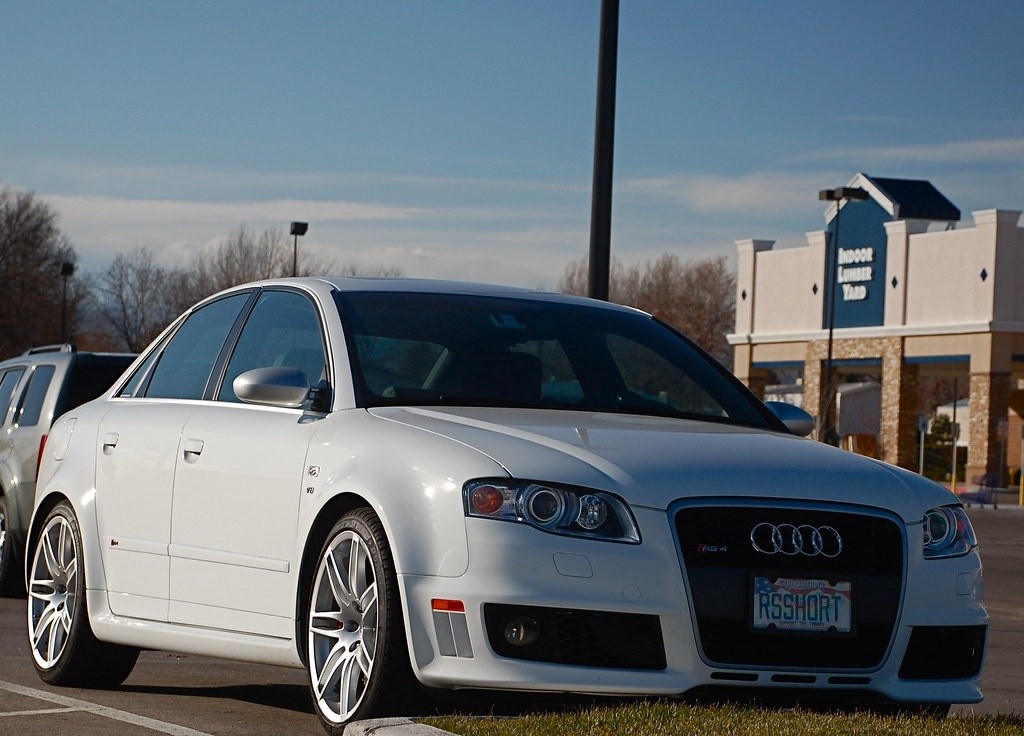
left=958, top=471, right=998, bottom=510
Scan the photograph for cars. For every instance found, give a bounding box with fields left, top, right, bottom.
left=24, top=278, right=989, bottom=736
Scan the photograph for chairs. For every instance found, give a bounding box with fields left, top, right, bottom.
left=476, top=349, right=541, bottom=399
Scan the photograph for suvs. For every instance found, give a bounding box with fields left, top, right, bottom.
left=0, top=344, right=140, bottom=598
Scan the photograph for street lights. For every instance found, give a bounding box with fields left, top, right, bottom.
left=290, top=221, right=309, bottom=277
left=819, top=186, right=870, bottom=443
left=60, top=261, right=74, bottom=344
